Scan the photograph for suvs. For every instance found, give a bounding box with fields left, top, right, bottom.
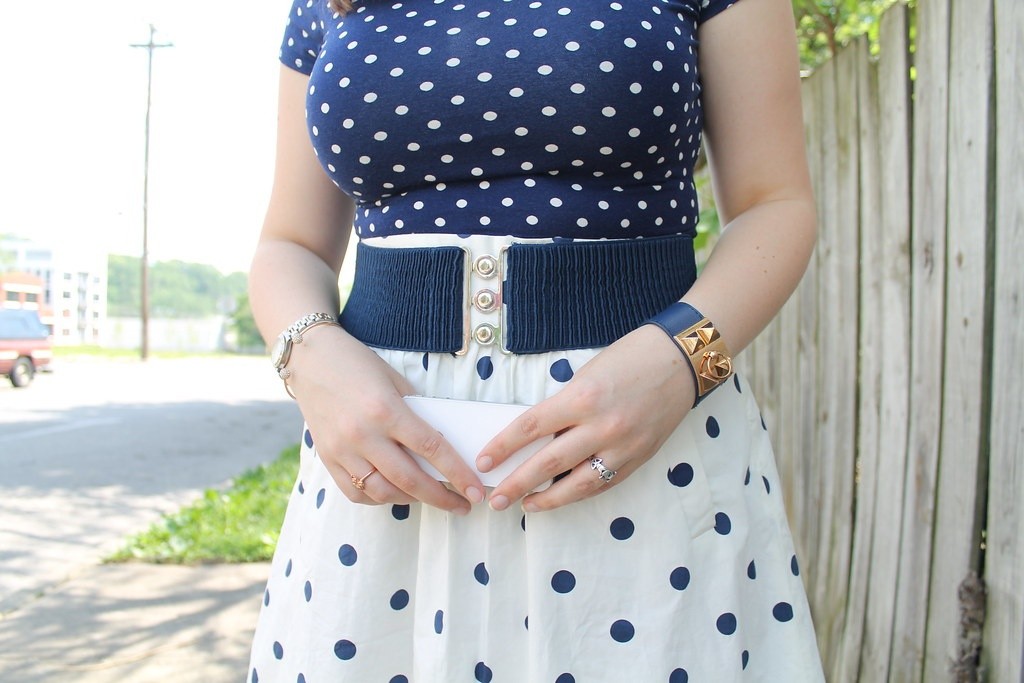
left=0, top=307, right=53, bottom=389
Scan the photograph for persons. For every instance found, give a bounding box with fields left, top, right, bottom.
left=249, top=0, right=829, bottom=683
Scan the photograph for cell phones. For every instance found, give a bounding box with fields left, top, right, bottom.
left=403, top=395, right=556, bottom=493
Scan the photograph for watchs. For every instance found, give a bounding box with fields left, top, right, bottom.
left=270, top=313, right=338, bottom=373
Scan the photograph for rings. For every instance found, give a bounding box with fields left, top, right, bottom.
left=351, top=468, right=376, bottom=490
left=588, top=455, right=618, bottom=482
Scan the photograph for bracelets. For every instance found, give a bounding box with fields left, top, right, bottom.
left=281, top=322, right=340, bottom=400
left=644, top=303, right=733, bottom=408
